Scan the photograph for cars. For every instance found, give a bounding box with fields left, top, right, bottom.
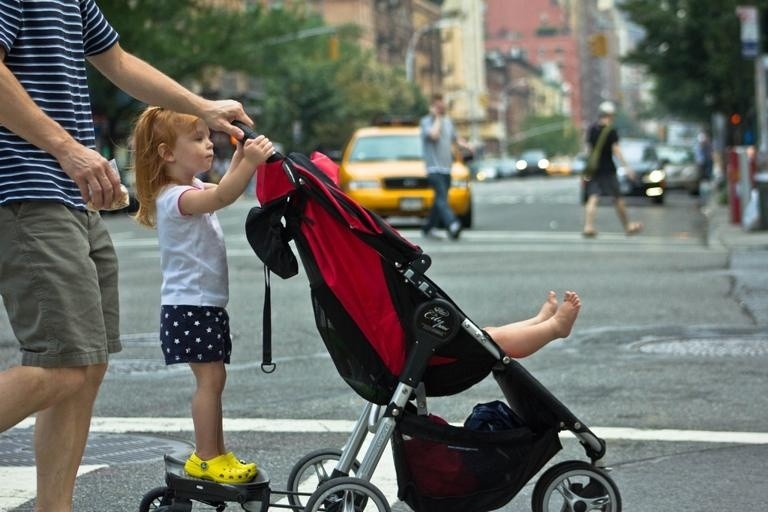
left=470, top=139, right=699, bottom=204
left=340, top=125, right=473, bottom=229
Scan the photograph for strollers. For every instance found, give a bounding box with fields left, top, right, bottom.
left=140, top=120, right=621, bottom=512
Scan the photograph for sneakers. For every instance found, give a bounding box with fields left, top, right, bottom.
left=425, top=222, right=463, bottom=238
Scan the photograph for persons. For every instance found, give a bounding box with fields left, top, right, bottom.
left=0, top=2, right=254, bottom=512
left=582, top=100, right=643, bottom=237
left=480, top=290, right=581, bottom=361
left=125, top=103, right=278, bottom=485
left=417, top=91, right=468, bottom=240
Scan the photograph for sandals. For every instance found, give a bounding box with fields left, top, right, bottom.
left=184, top=451, right=256, bottom=484
